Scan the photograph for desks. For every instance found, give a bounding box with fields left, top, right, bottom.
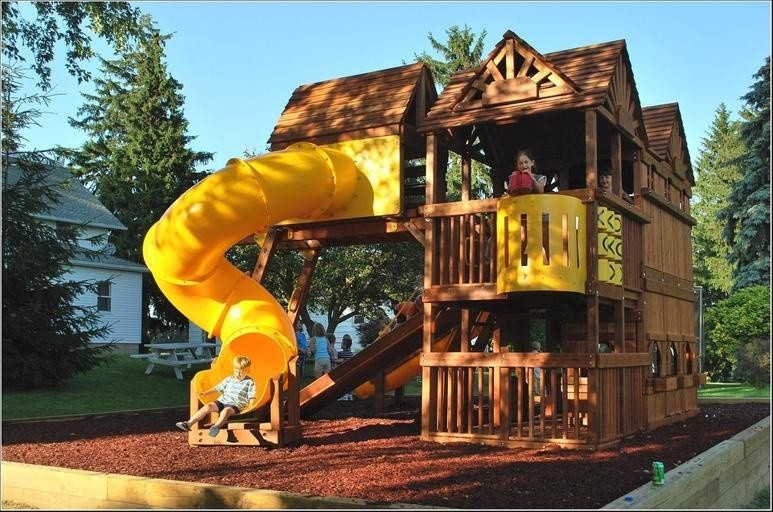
left=143, top=342, right=220, bottom=380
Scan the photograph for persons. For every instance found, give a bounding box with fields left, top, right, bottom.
left=294, top=321, right=354, bottom=401
left=507, top=149, right=547, bottom=195
left=530, top=340, right=542, bottom=395
left=174, top=355, right=257, bottom=438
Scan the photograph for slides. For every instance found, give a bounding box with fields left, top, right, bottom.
left=141, top=143, right=358, bottom=417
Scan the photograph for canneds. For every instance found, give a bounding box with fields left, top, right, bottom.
left=652, top=461, right=665, bottom=486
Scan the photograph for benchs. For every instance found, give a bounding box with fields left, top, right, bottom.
left=167, top=358, right=214, bottom=365
left=129, top=352, right=189, bottom=359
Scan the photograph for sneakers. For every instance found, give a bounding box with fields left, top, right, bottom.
left=175, top=420, right=193, bottom=432
left=207, top=425, right=220, bottom=437
